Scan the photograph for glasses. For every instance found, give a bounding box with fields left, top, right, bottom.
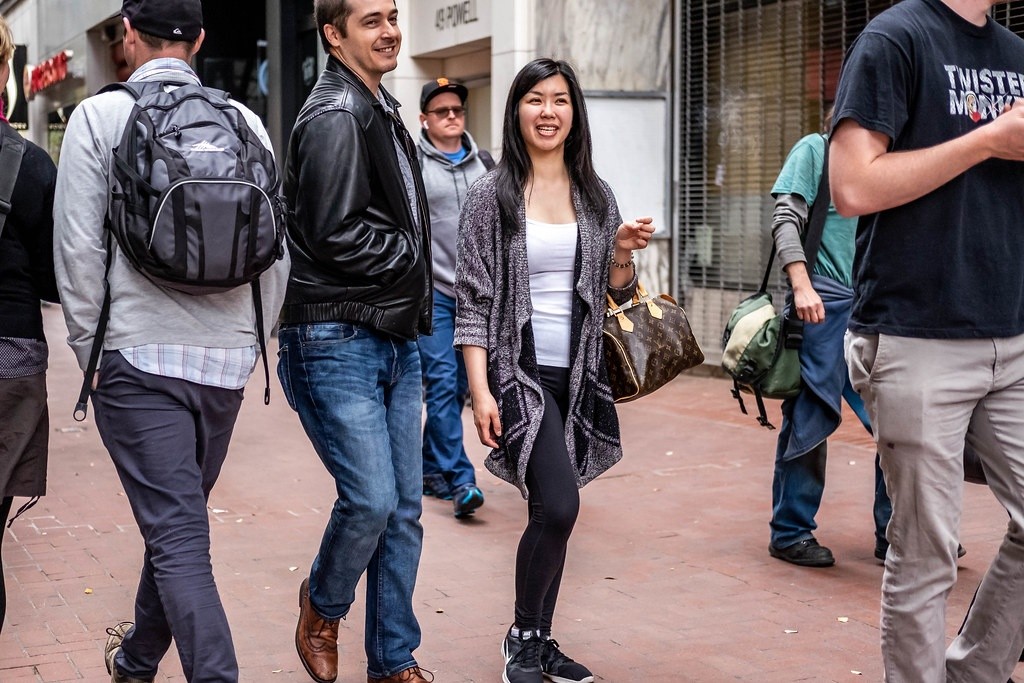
left=427, top=106, right=467, bottom=118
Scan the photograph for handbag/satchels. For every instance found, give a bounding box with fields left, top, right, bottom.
left=605, top=279, right=705, bottom=405
left=721, top=291, right=801, bottom=430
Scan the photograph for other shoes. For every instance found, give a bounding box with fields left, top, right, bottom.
left=104, top=621, right=156, bottom=683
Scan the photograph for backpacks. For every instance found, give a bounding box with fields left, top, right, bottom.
left=71, top=80, right=288, bottom=422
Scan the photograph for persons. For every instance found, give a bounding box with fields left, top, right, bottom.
left=829, top=0, right=1024, bottom=683
left=52, top=0, right=290, bottom=683
left=771, top=106, right=968, bottom=567
left=454, top=57, right=656, bottom=683
left=276, top=0, right=435, bottom=683
left=417, top=78, right=501, bottom=518
left=0, top=12, right=62, bottom=632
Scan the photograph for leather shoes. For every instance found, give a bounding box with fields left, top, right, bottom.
left=366, top=666, right=435, bottom=683
left=874, top=534, right=970, bottom=564
left=769, top=539, right=835, bottom=565
left=295, top=577, right=347, bottom=683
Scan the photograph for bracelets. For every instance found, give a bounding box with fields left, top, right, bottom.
left=611, top=250, right=634, bottom=268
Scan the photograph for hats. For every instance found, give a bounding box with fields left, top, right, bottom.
left=121, top=0, right=203, bottom=42
left=420, top=78, right=468, bottom=114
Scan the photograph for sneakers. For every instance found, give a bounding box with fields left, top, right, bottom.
left=501, top=622, right=543, bottom=683
left=454, top=485, right=485, bottom=517
left=423, top=475, right=455, bottom=500
left=537, top=635, right=594, bottom=683
